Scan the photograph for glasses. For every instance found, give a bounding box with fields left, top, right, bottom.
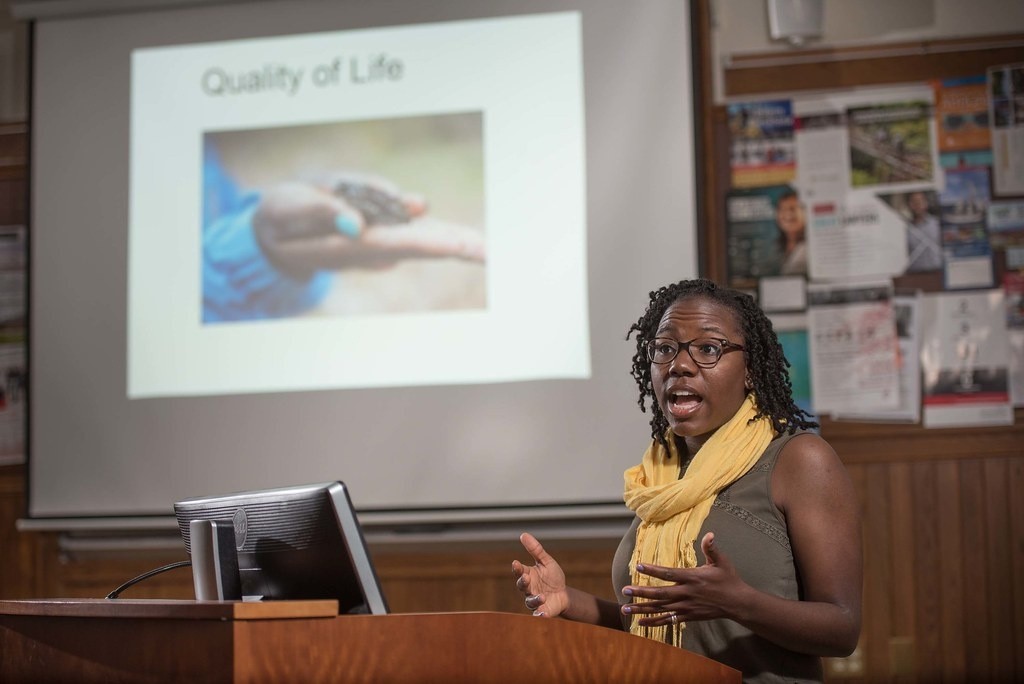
left=647, top=337, right=748, bottom=365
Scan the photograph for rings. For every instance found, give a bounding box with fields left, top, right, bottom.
left=525, top=596, right=537, bottom=610
left=670, top=613, right=678, bottom=626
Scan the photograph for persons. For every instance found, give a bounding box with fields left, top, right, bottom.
left=763, top=186, right=807, bottom=274
left=906, top=192, right=940, bottom=265
left=201, top=134, right=487, bottom=327
left=512, top=278, right=860, bottom=684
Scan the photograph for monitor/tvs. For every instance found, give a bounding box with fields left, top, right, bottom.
left=173, top=479, right=391, bottom=614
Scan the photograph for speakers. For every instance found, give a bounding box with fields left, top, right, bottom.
left=765, top=0, right=825, bottom=42
